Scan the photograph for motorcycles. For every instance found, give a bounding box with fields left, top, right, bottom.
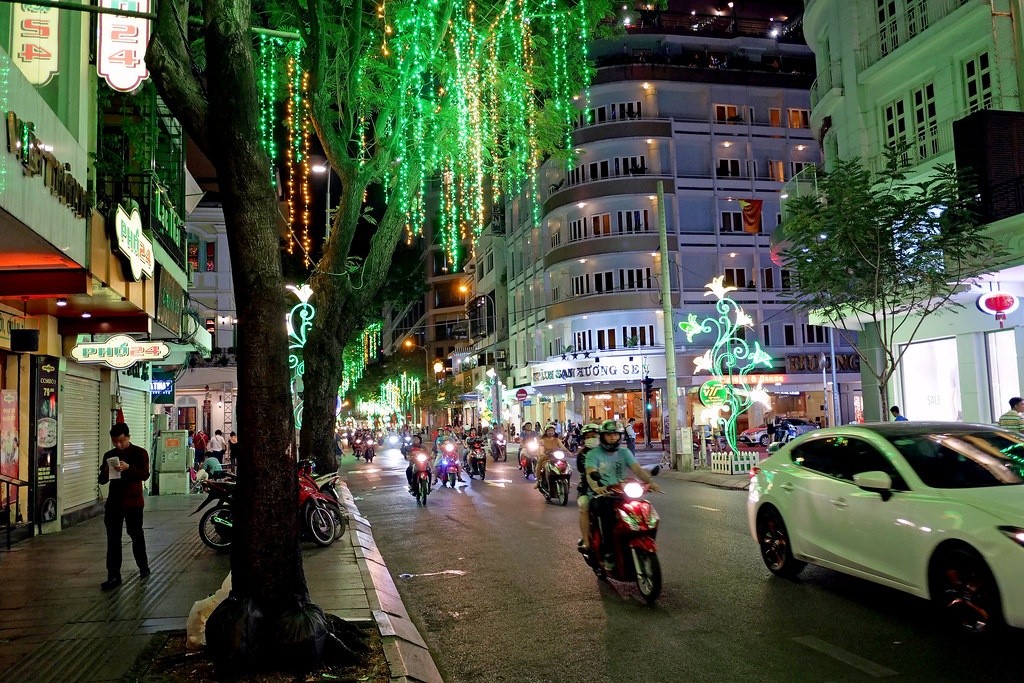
left=577, top=465, right=665, bottom=603
left=195, top=455, right=346, bottom=553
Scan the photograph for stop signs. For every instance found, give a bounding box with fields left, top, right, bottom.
left=515, top=389, right=527, bottom=400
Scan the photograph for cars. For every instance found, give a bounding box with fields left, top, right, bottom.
left=747, top=422, right=1024, bottom=646
left=739, top=419, right=824, bottom=448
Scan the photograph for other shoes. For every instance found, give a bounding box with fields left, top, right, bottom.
left=534, top=482, right=540, bottom=490
left=101, top=576, right=122, bottom=590
left=139, top=562, right=151, bottom=577
left=411, top=490, right=416, bottom=496
left=578, top=547, right=590, bottom=554
left=457, top=478, right=466, bottom=482
left=519, top=466, right=523, bottom=470
left=603, top=553, right=616, bottom=570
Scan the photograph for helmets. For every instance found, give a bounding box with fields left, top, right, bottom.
left=436, top=428, right=443, bottom=431
left=413, top=433, right=422, bottom=441
left=599, top=420, right=625, bottom=452
left=545, top=425, right=555, bottom=431
left=581, top=423, right=599, bottom=433
left=444, top=427, right=451, bottom=433
left=523, top=422, right=532, bottom=428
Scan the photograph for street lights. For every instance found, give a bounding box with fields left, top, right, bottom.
left=405, top=340, right=431, bottom=427
left=459, top=286, right=501, bottom=428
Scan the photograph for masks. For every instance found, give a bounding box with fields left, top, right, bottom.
left=585, top=437, right=600, bottom=448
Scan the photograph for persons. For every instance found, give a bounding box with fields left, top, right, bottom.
left=346, top=428, right=374, bottom=454
left=510, top=422, right=516, bottom=443
left=377, top=429, right=384, bottom=436
left=890, top=406, right=908, bottom=421
left=189, top=430, right=237, bottom=490
left=491, top=423, right=501, bottom=453
left=98, top=423, right=150, bottom=587
left=535, top=418, right=571, bottom=434
left=534, top=425, right=572, bottom=489
left=765, top=411, right=776, bottom=452
left=624, top=417, right=639, bottom=455
left=431, top=424, right=466, bottom=485
left=388, top=428, right=397, bottom=436
left=462, top=427, right=487, bottom=473
left=705, top=423, right=723, bottom=452
left=406, top=435, right=432, bottom=492
left=576, top=420, right=663, bottom=555
left=453, top=423, right=471, bottom=432
left=998, top=396, right=1024, bottom=433
left=335, top=430, right=345, bottom=469
left=518, top=422, right=537, bottom=470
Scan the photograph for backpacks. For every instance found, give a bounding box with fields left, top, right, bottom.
left=623, top=425, right=633, bottom=442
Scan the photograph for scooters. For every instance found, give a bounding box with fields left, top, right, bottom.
left=489, top=429, right=508, bottom=462
left=352, top=431, right=414, bottom=463
left=520, top=441, right=539, bottom=480
left=537, top=440, right=576, bottom=507
left=406, top=453, right=433, bottom=505
left=436, top=442, right=460, bottom=488
left=462, top=440, right=488, bottom=480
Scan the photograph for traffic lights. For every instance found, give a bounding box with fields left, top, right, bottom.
left=647, top=378, right=655, bottom=399
